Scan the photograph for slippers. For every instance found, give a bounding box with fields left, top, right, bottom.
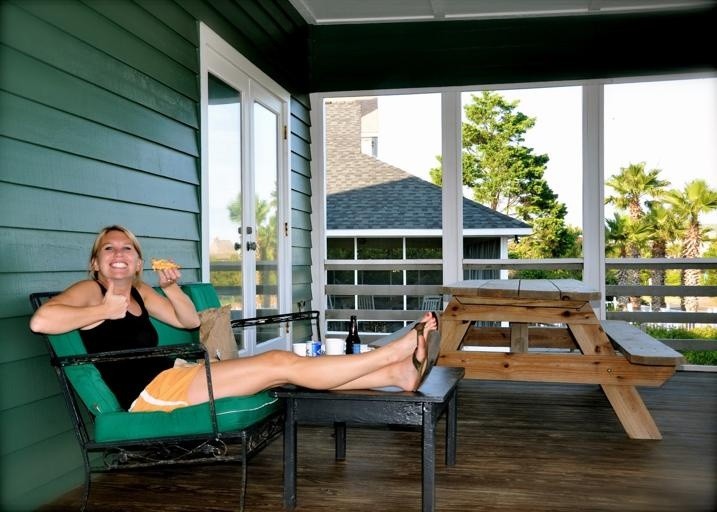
left=410, top=311, right=442, bottom=345
left=412, top=330, right=440, bottom=393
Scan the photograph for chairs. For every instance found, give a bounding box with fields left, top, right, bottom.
left=358, top=295, right=376, bottom=310
left=422, top=295, right=441, bottom=312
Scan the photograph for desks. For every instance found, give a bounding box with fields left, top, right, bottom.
left=268, top=362, right=467, bottom=512
left=431, top=277, right=663, bottom=442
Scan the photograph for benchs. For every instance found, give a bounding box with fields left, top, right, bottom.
left=600, top=318, right=685, bottom=388
left=367, top=318, right=418, bottom=351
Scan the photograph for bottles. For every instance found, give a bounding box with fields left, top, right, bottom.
left=347, top=315, right=361, bottom=357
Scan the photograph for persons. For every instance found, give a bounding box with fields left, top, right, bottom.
left=29, top=225, right=440, bottom=413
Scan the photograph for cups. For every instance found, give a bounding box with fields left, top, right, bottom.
left=325, top=338, right=344, bottom=356
left=354, top=344, right=368, bottom=353
left=294, top=343, right=305, bottom=357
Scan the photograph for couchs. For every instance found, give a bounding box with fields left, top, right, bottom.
left=29, top=279, right=322, bottom=511
